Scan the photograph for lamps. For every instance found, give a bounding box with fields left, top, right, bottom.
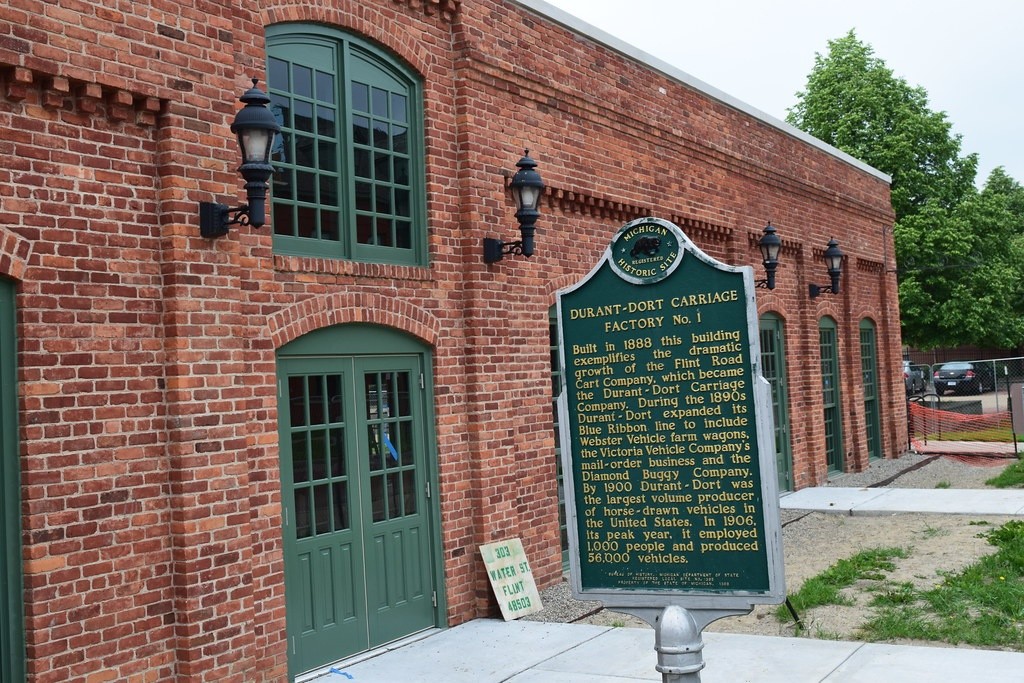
left=484, top=148, right=545, bottom=263
left=808, top=235, right=843, bottom=299
left=199, top=73, right=280, bottom=238
left=754, top=221, right=781, bottom=290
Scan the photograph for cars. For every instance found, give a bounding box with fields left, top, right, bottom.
left=933, top=361, right=998, bottom=395
left=903, top=361, right=927, bottom=395
